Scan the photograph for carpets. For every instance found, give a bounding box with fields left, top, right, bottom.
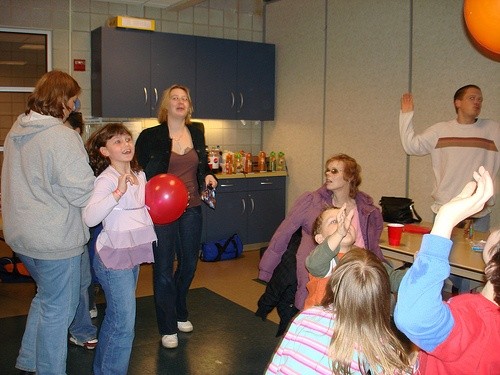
left=0, top=287, right=283, bottom=375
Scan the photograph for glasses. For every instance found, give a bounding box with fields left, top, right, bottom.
left=324, top=168, right=344, bottom=174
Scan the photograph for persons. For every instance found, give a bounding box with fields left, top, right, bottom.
left=1, top=69, right=97, bottom=375
left=134, top=84, right=218, bottom=347
left=257, top=154, right=500, bottom=375
left=81, top=123, right=190, bottom=375
left=397, top=84, right=500, bottom=233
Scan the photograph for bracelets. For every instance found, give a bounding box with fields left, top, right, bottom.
left=115, top=188, right=124, bottom=197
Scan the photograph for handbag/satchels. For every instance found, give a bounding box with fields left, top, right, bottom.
left=379, top=196, right=422, bottom=224
left=200, top=233, right=243, bottom=262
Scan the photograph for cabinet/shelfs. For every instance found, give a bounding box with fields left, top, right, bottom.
left=91, top=26, right=275, bottom=120
left=200, top=177, right=286, bottom=246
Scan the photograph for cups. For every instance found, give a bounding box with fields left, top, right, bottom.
left=386, top=223, right=404, bottom=246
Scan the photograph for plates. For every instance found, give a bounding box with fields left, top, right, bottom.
left=404, top=224, right=431, bottom=234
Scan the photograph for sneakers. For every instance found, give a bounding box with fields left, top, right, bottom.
left=177, top=320, right=193, bottom=332
left=88, top=306, right=97, bottom=318
left=69, top=337, right=98, bottom=349
left=161, top=333, right=178, bottom=348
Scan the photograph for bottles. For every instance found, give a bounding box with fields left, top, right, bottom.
left=258, top=151, right=266, bottom=171
left=269, top=151, right=276, bottom=171
left=205, top=145, right=252, bottom=174
left=276, top=152, right=287, bottom=171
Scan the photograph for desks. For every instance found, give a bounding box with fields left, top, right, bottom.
left=379, top=220, right=491, bottom=294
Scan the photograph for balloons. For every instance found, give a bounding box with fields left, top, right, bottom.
left=145, top=174, right=188, bottom=224
left=464, top=0, right=500, bottom=54
